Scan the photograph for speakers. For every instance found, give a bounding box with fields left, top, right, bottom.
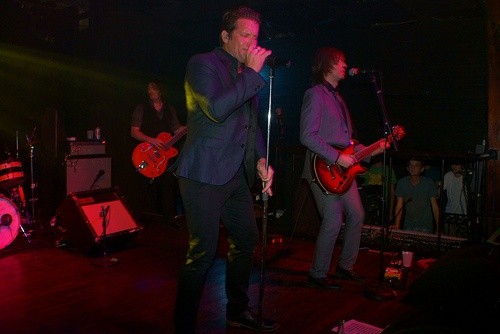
left=60, top=157, right=111, bottom=198
left=63, top=190, right=139, bottom=257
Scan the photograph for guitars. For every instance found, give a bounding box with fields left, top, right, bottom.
left=131, top=125, right=191, bottom=178
left=311, top=125, right=407, bottom=196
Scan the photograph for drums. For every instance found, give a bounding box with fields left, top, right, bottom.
left=0, top=193, right=21, bottom=251
left=0, top=159, right=24, bottom=189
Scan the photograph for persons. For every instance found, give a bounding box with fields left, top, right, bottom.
left=390, top=157, right=442, bottom=236
left=132, top=77, right=187, bottom=226
left=170, top=7, right=278, bottom=334
left=297, top=48, right=390, bottom=288
left=444, top=159, right=467, bottom=214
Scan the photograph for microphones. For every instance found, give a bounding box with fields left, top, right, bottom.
left=266, top=54, right=289, bottom=67
left=347, top=68, right=377, bottom=76
left=95, top=170, right=105, bottom=182
left=100, top=205, right=110, bottom=217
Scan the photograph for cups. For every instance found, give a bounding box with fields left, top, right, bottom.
left=403, top=252, right=413, bottom=267
left=87, top=130, right=93, bottom=139
left=95, top=129, right=100, bottom=139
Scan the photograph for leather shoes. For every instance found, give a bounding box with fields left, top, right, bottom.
left=225, top=303, right=280, bottom=331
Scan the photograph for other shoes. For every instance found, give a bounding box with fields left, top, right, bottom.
left=335, top=265, right=365, bottom=281
left=165, top=216, right=183, bottom=229
left=308, top=276, right=342, bottom=290
